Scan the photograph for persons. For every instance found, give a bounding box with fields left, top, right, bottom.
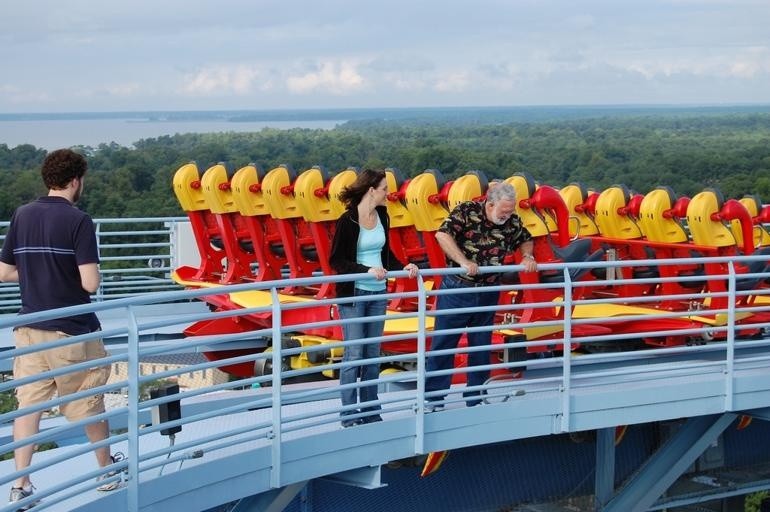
left=328, top=169, right=419, bottom=428
left=0, top=147, right=129, bottom=512
left=417, top=183, right=538, bottom=412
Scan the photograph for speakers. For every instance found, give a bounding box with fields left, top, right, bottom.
left=158, top=384, right=182, bottom=436
left=505, top=333, right=528, bottom=372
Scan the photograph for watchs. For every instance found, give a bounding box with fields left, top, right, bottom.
left=523, top=255, right=535, bottom=262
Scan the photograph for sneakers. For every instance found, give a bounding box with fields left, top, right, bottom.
left=9, top=483, right=42, bottom=511
left=96, top=452, right=132, bottom=491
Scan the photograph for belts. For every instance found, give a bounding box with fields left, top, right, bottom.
left=453, top=276, right=483, bottom=287
